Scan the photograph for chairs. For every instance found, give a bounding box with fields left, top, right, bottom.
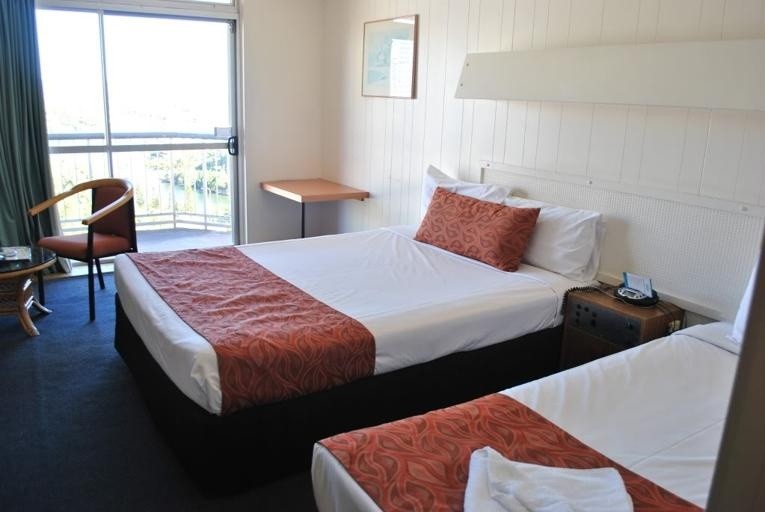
left=28, top=178, right=139, bottom=321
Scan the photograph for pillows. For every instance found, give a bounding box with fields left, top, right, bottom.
left=732, top=260, right=760, bottom=344
left=417, top=165, right=513, bottom=224
left=413, top=187, right=541, bottom=273
left=503, top=195, right=606, bottom=282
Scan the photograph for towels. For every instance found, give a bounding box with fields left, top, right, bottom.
left=464, top=445, right=634, bottom=512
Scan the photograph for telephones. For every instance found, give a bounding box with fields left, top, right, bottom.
left=614, top=283, right=658, bottom=307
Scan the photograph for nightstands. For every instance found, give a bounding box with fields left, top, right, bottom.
left=560, top=286, right=684, bottom=370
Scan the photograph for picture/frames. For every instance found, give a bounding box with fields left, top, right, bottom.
left=361, top=14, right=418, bottom=99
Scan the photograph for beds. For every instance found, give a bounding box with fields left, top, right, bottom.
left=311, top=322, right=741, bottom=512
left=113, top=223, right=608, bottom=496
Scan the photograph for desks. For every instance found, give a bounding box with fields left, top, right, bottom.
left=260, top=178, right=369, bottom=237
